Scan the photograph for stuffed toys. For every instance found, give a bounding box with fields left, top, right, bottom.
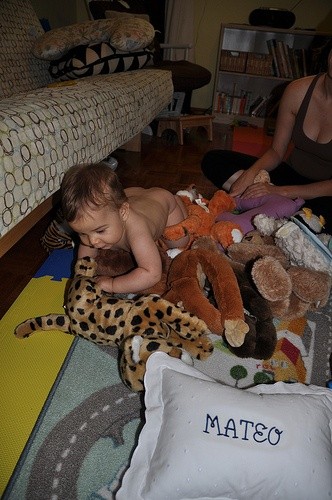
left=158, top=189, right=244, bottom=253
left=205, top=256, right=276, bottom=361
left=42, top=207, right=75, bottom=254
left=13, top=255, right=216, bottom=393
left=94, top=240, right=251, bottom=347
left=224, top=216, right=330, bottom=317
left=252, top=212, right=332, bottom=277
left=292, top=206, right=325, bottom=236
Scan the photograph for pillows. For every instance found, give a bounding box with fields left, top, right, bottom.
left=49, top=41, right=154, bottom=82
left=115, top=351, right=332, bottom=499
left=32, top=17, right=154, bottom=61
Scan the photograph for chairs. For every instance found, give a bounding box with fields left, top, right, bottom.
left=105, top=11, right=212, bottom=113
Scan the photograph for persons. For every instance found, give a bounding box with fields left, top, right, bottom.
left=199, top=50, right=332, bottom=236
left=62, top=162, right=192, bottom=295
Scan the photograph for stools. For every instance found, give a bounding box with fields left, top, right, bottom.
left=157, top=114, right=216, bottom=145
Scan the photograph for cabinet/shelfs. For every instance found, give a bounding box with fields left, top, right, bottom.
left=212, top=23, right=332, bottom=129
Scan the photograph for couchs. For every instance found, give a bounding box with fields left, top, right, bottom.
left=0, top=0, right=174, bottom=257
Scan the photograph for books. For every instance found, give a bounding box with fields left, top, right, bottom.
left=212, top=90, right=281, bottom=121
left=264, top=38, right=327, bottom=81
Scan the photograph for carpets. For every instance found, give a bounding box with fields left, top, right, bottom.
left=1, top=296, right=330, bottom=499
